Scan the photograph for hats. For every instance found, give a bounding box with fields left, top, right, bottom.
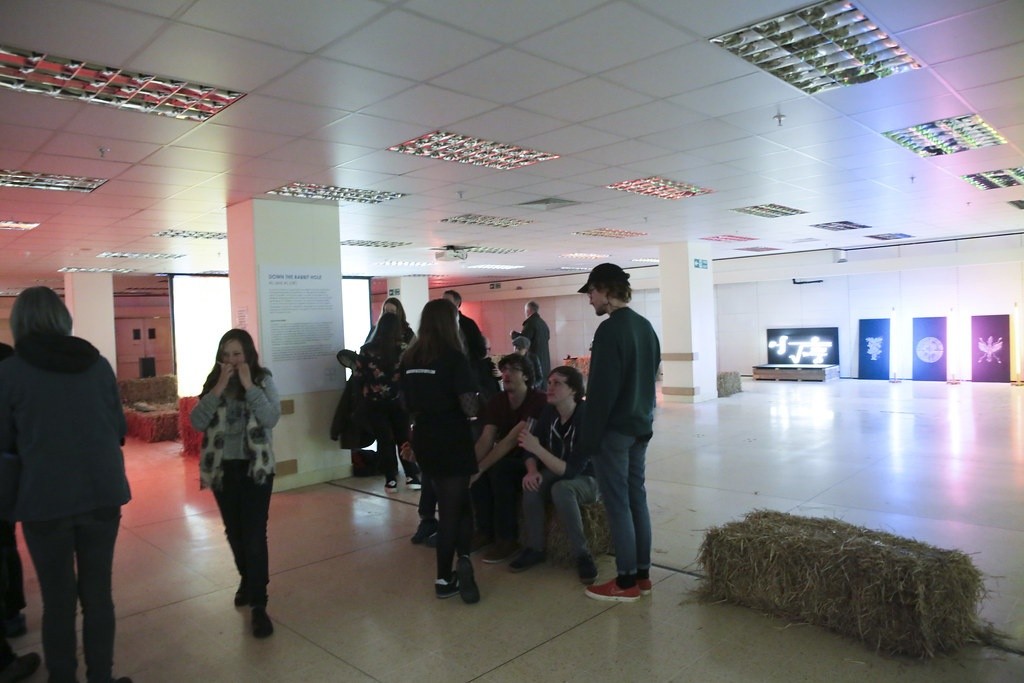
left=578, top=262, right=626, bottom=294
left=512, top=337, right=530, bottom=348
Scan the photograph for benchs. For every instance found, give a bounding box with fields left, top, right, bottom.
left=514, top=492, right=610, bottom=558
left=695, top=511, right=984, bottom=659
left=716, top=372, right=742, bottom=397
left=117, top=371, right=180, bottom=443
left=177, top=395, right=203, bottom=453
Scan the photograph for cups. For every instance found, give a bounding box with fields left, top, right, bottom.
left=522, top=416, right=538, bottom=434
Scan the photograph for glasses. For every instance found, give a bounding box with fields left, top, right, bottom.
left=500, top=366, right=524, bottom=373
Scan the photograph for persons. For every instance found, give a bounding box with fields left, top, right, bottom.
left=191, top=330, right=281, bottom=639
left=510, top=299, right=552, bottom=393
left=577, top=263, right=661, bottom=603
left=468, top=354, right=552, bottom=564
left=353, top=311, right=422, bottom=494
left=443, top=289, right=487, bottom=362
left=399, top=299, right=482, bottom=604
left=0, top=286, right=132, bottom=683
left=509, top=367, right=602, bottom=584
left=362, top=298, right=417, bottom=398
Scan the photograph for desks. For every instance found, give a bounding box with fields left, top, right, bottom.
left=752, top=364, right=840, bottom=382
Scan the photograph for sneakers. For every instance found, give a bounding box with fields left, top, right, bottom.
left=509, top=551, right=545, bottom=571
left=435, top=570, right=461, bottom=598
left=578, top=555, right=599, bottom=583
left=585, top=578, right=640, bottom=602
left=457, top=556, right=478, bottom=603
left=638, top=578, right=652, bottom=596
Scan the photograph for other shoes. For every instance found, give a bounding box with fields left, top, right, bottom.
left=234, top=586, right=247, bottom=605
left=2, top=652, right=40, bottom=682
left=252, top=607, right=271, bottom=637
left=405, top=475, right=422, bottom=490
left=384, top=480, right=396, bottom=493
left=412, top=518, right=439, bottom=543
left=471, top=536, right=495, bottom=553
left=481, top=541, right=518, bottom=563
